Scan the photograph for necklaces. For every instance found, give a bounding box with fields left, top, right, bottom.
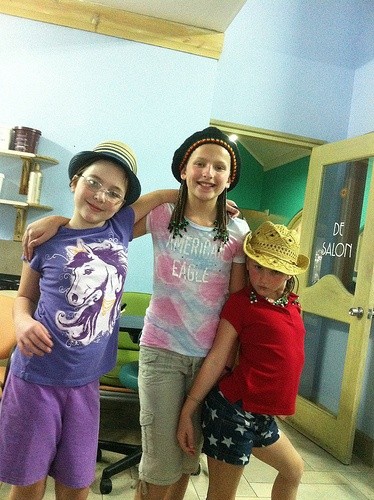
left=262, top=293, right=289, bottom=308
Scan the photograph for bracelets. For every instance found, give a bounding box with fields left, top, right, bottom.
left=187, top=395, right=202, bottom=405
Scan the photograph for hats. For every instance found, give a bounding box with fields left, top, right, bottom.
left=172, top=127, right=240, bottom=192
left=67, top=139, right=141, bottom=208
left=243, top=221, right=310, bottom=275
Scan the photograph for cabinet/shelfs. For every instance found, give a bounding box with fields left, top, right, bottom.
left=0, top=147, right=59, bottom=242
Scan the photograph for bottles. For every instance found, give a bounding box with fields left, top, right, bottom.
left=27, top=164, right=43, bottom=204
left=14, top=208, right=27, bottom=242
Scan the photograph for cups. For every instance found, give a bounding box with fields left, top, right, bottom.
left=13, top=126, right=41, bottom=154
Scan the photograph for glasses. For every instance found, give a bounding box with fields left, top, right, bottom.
left=80, top=173, right=127, bottom=205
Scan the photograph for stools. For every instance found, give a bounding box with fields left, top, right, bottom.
left=96, top=361, right=201, bottom=495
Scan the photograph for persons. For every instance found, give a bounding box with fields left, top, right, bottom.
left=1, top=141, right=251, bottom=500
left=176, top=222, right=312, bottom=500
left=21, top=126, right=251, bottom=500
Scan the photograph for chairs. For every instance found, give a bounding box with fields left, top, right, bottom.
left=99, top=291, right=152, bottom=403
left=0, top=294, right=18, bottom=403
